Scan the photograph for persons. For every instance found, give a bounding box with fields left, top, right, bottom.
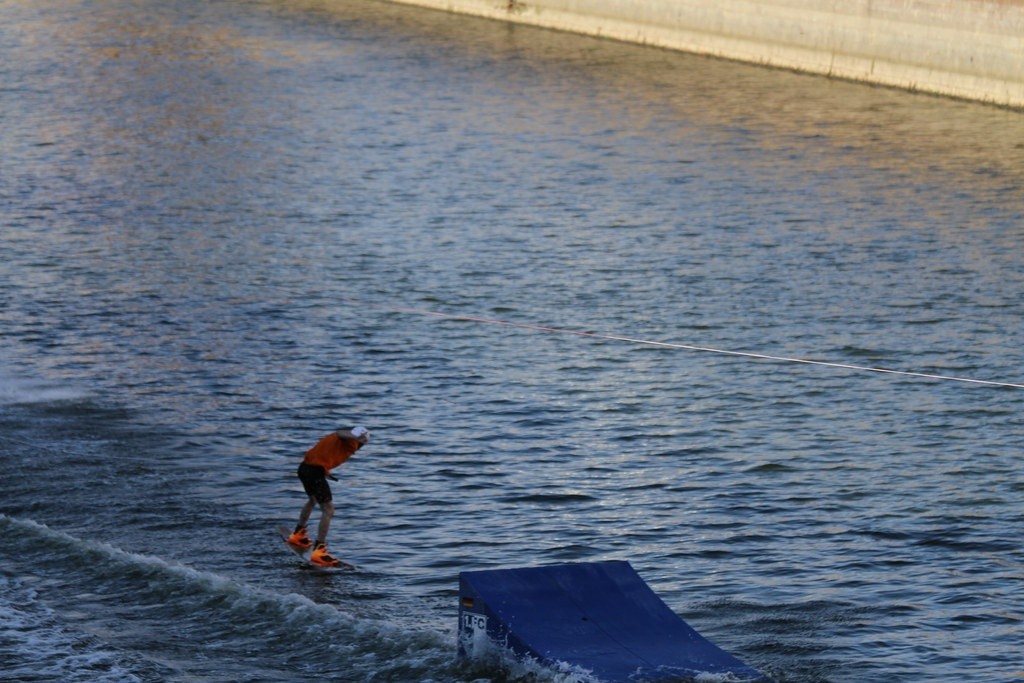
left=287, top=426, right=370, bottom=568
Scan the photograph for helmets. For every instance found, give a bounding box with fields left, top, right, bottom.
left=351, top=427, right=369, bottom=444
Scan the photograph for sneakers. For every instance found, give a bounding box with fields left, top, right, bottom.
left=288, top=528, right=312, bottom=548
left=310, top=543, right=338, bottom=564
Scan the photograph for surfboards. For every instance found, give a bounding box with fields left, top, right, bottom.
left=277, top=525, right=353, bottom=571
